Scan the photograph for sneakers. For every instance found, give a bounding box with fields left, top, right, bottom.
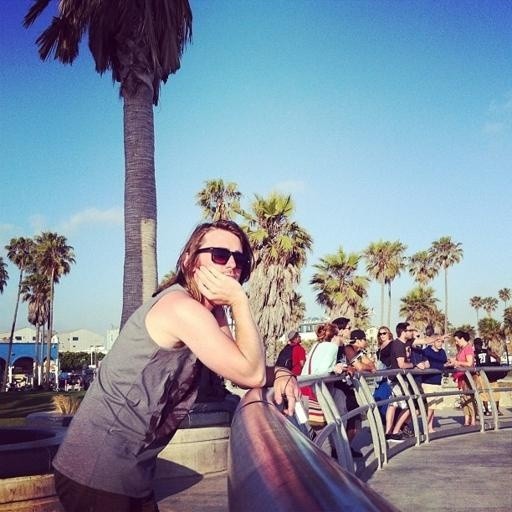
left=351, top=447, right=363, bottom=457
left=386, top=423, right=413, bottom=443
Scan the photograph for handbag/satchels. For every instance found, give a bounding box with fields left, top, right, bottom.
left=300, top=386, right=326, bottom=427
left=374, top=360, right=386, bottom=382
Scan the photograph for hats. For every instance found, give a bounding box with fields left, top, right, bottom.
left=332, top=318, right=350, bottom=330
left=350, top=330, right=366, bottom=340
left=288, top=330, right=296, bottom=341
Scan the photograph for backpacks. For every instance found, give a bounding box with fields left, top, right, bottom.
left=276, top=344, right=291, bottom=370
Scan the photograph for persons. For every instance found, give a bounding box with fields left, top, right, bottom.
left=50, top=218, right=301, bottom=511
left=268, top=317, right=504, bottom=462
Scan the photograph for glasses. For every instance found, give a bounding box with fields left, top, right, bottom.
left=196, top=247, right=250, bottom=272
left=404, top=329, right=412, bottom=332
left=379, top=332, right=387, bottom=335
